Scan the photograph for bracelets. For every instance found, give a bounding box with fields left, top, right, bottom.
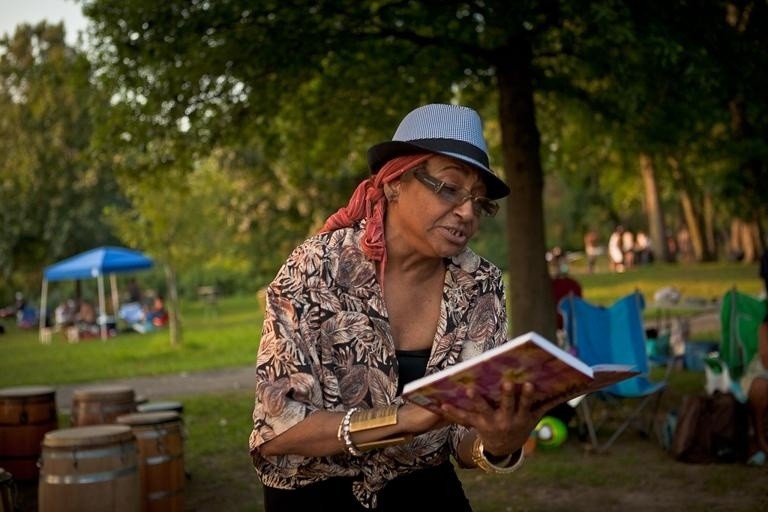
left=472, top=437, right=527, bottom=476
left=350, top=404, right=412, bottom=451
left=337, top=407, right=363, bottom=457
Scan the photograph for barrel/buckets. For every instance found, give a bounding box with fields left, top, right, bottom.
left=0, top=384, right=186, bottom=512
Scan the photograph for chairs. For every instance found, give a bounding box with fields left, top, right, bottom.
left=554, top=295, right=688, bottom=463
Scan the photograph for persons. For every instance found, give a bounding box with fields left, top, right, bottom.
left=542, top=213, right=692, bottom=277
left=248, top=105, right=643, bottom=511
left=2, top=274, right=169, bottom=341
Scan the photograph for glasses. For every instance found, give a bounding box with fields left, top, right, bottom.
left=414, top=168, right=500, bottom=219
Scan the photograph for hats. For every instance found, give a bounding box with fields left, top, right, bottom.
left=363, top=100, right=512, bottom=201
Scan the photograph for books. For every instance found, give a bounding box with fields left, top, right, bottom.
left=402, top=329, right=642, bottom=433
